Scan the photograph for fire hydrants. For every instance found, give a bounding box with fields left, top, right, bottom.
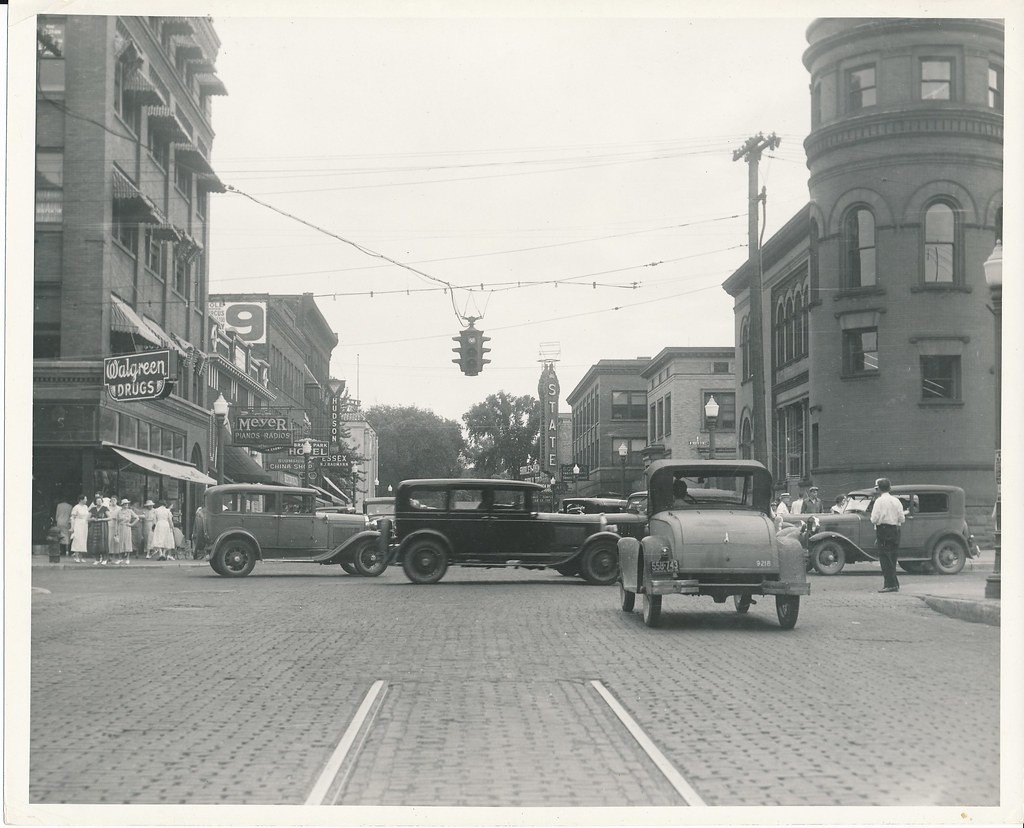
left=46, top=526, right=65, bottom=564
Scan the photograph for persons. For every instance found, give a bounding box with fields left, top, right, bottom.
left=801, top=487, right=823, bottom=514
left=870, top=478, right=905, bottom=595
left=673, top=481, right=690, bottom=505
left=477, top=491, right=515, bottom=509
left=54, top=491, right=176, bottom=566
left=829, top=495, right=846, bottom=514
left=191, top=507, right=206, bottom=560
left=789, top=493, right=804, bottom=515
left=777, top=493, right=792, bottom=514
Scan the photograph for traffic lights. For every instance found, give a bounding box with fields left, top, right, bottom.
left=452, top=331, right=464, bottom=372
left=463, top=332, right=479, bottom=373
left=477, top=330, right=492, bottom=371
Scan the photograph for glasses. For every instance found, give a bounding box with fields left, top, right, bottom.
left=875, top=487, right=878, bottom=490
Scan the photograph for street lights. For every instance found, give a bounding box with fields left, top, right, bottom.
left=374, top=478, right=380, bottom=497
left=618, top=442, right=628, bottom=500
left=704, top=394, right=720, bottom=490
left=550, top=476, right=557, bottom=513
left=302, top=441, right=312, bottom=512
left=984, top=239, right=1000, bottom=602
left=213, top=392, right=229, bottom=484
left=571, top=463, right=580, bottom=498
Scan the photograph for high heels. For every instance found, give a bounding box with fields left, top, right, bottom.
left=156, top=554, right=163, bottom=561
left=81, top=559, right=86, bottom=563
left=101, top=560, right=108, bottom=565
left=115, top=559, right=123, bottom=564
left=93, top=561, right=100, bottom=565
left=71, top=553, right=75, bottom=557
left=167, top=555, right=175, bottom=561
left=162, top=555, right=166, bottom=561
left=74, top=559, right=80, bottom=563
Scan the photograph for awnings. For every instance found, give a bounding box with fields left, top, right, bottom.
left=224, top=428, right=273, bottom=483
left=324, top=476, right=352, bottom=502
left=110, top=294, right=187, bottom=358
left=309, top=484, right=345, bottom=505
left=111, top=447, right=217, bottom=485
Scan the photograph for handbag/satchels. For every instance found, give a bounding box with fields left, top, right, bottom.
left=114, top=535, right=120, bottom=543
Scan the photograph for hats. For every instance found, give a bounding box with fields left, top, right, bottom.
left=144, top=500, right=155, bottom=506
left=781, top=493, right=790, bottom=496
left=810, top=487, right=818, bottom=492
left=121, top=499, right=130, bottom=505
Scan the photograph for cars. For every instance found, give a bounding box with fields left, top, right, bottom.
left=385, top=478, right=623, bottom=586
left=782, top=477, right=981, bottom=576
left=191, top=483, right=391, bottom=579
left=616, top=458, right=812, bottom=631
left=352, top=492, right=753, bottom=552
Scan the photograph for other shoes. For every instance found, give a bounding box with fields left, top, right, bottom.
left=125, top=560, right=130, bottom=564
left=65, top=550, right=69, bottom=555
left=879, top=586, right=899, bottom=593
left=146, top=555, right=149, bottom=559
